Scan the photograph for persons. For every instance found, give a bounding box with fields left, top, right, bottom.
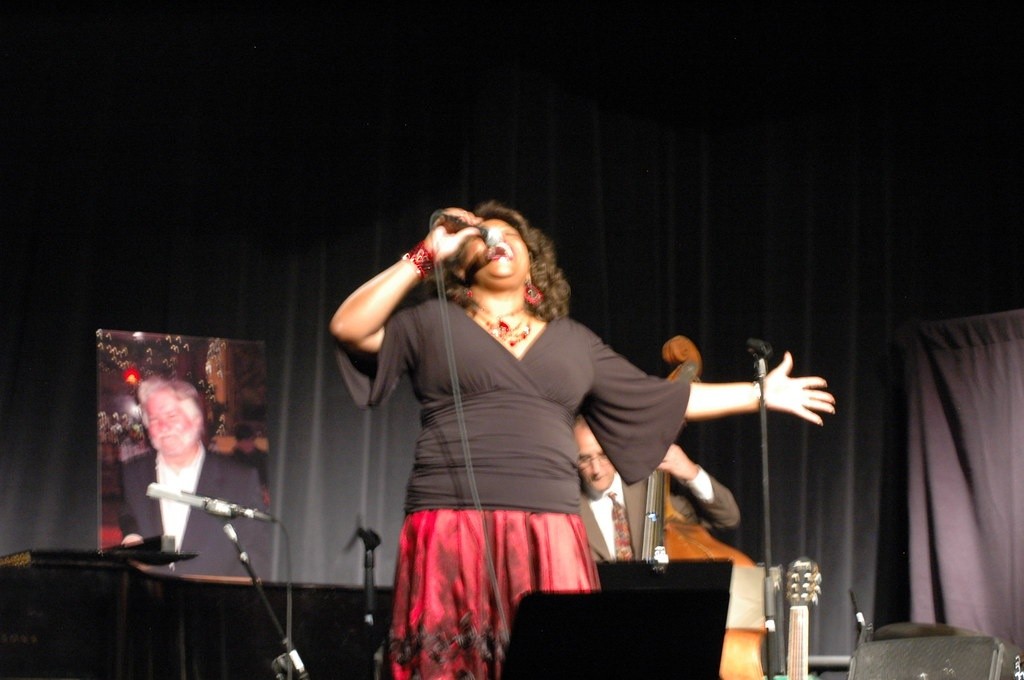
left=562, top=407, right=741, bottom=563
left=330, top=203, right=836, bottom=680
left=119, top=375, right=267, bottom=579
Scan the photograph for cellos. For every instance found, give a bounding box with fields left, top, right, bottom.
left=642, top=334, right=768, bottom=680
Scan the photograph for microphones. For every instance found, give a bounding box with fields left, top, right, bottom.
left=438, top=211, right=503, bottom=248
left=145, top=482, right=271, bottom=527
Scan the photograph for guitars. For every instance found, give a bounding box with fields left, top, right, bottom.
left=786, top=557, right=821, bottom=680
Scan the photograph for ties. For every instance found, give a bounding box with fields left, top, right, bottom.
left=607, top=491, right=634, bottom=561
